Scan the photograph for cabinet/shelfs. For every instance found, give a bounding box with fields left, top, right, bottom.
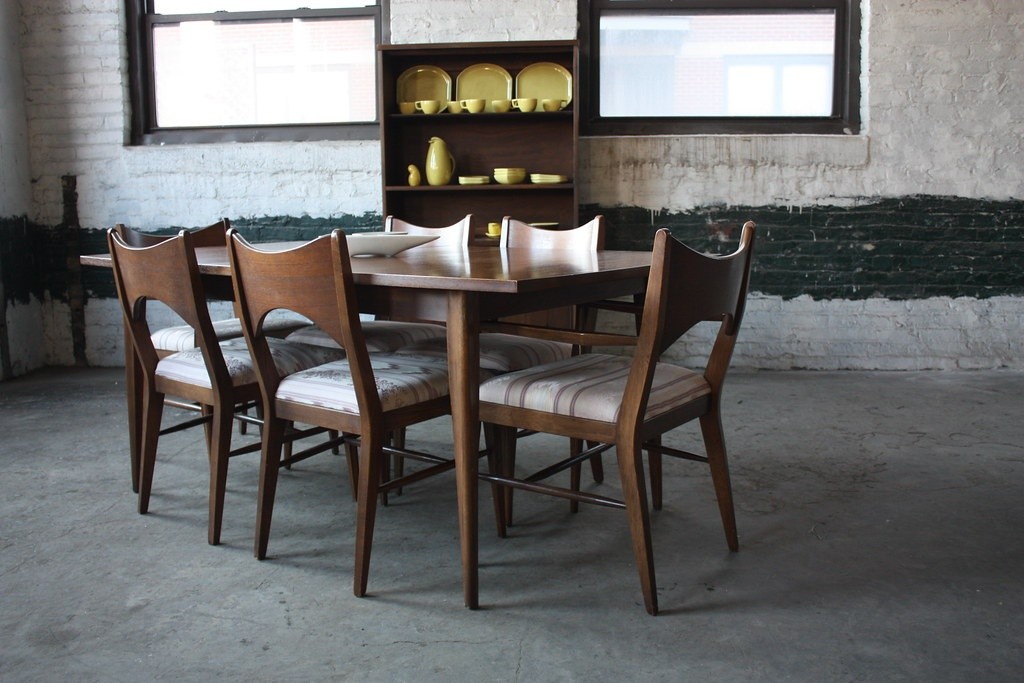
left=375, top=39, right=578, bottom=245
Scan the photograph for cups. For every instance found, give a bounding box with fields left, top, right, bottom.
left=400, top=102, right=415, bottom=114
left=488, top=223, right=501, bottom=234
left=512, top=99, right=537, bottom=113
left=446, top=101, right=464, bottom=114
left=491, top=100, right=515, bottom=113
left=460, top=99, right=485, bottom=114
left=415, top=100, right=439, bottom=114
left=542, top=99, right=567, bottom=112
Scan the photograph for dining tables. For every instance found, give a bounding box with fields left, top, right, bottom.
left=81, top=240, right=655, bottom=607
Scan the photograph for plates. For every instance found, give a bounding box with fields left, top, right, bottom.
left=316, top=230, right=442, bottom=257
left=485, top=233, right=501, bottom=238
left=530, top=174, right=568, bottom=184
left=493, top=168, right=525, bottom=185
left=396, top=65, right=452, bottom=114
left=515, top=62, right=572, bottom=112
left=458, top=176, right=490, bottom=185
left=456, top=63, right=512, bottom=113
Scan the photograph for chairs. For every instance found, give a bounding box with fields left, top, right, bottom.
left=228, top=231, right=506, bottom=600
left=113, top=213, right=607, bottom=487
left=106, top=226, right=360, bottom=545
left=478, top=219, right=758, bottom=615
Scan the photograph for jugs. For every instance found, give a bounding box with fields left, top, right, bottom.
left=425, top=136, right=455, bottom=185
